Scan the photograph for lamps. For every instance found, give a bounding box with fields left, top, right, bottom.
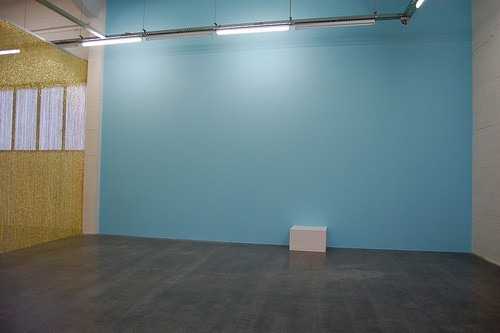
left=295, top=19, right=376, bottom=30
left=416, top=0, right=425, bottom=8
left=82, top=36, right=146, bottom=47
left=216, top=23, right=293, bottom=36
left=0, top=47, right=20, bottom=55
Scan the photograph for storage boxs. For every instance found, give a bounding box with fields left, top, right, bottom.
left=289, top=225, right=327, bottom=252
